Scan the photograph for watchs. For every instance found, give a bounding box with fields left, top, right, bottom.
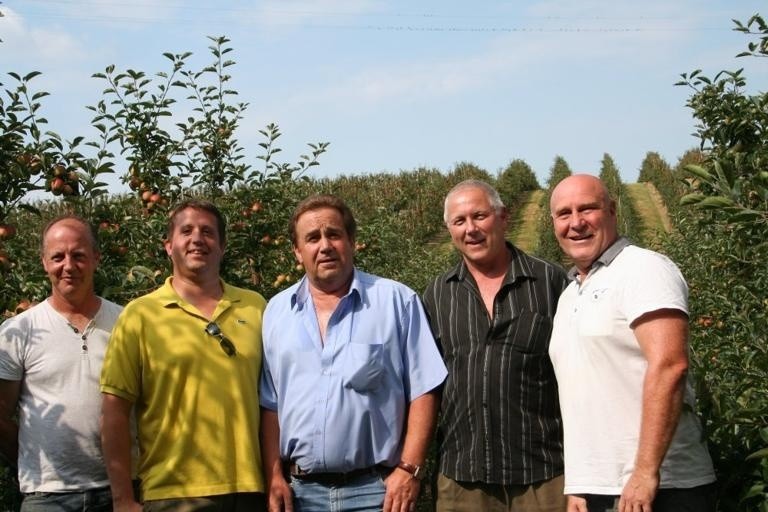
left=397, top=462, right=426, bottom=480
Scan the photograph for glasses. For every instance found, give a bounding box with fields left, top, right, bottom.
left=205, top=320, right=236, bottom=356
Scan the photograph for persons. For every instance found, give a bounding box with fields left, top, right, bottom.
left=0, top=214, right=141, bottom=512
left=98, top=200, right=268, bottom=512
left=425, top=179, right=568, bottom=512
left=542, top=172, right=719, bottom=512
left=258, top=191, right=447, bottom=512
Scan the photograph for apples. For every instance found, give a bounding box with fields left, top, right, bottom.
left=710, top=353, right=718, bottom=363
left=1, top=300, right=38, bottom=318
left=14, top=150, right=83, bottom=202
left=271, top=261, right=304, bottom=292
left=696, top=309, right=725, bottom=331
left=354, top=234, right=380, bottom=263
left=0, top=225, right=18, bottom=242
left=97, top=219, right=131, bottom=262
left=119, top=172, right=170, bottom=213
left=232, top=202, right=286, bottom=251
left=0, top=256, right=9, bottom=272
left=218, top=128, right=226, bottom=137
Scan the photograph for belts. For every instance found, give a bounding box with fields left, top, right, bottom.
left=283, top=462, right=308, bottom=476
